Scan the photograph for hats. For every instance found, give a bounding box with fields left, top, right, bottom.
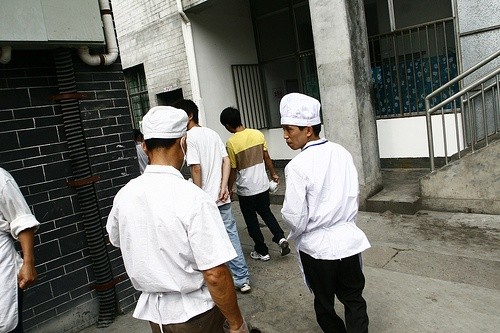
left=279, top=92, right=321, bottom=126
left=142, top=106, right=190, bottom=140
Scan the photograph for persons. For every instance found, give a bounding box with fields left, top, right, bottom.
left=280, top=92, right=372, bottom=333
left=0, top=167, right=40, bottom=333
left=220, top=107, right=291, bottom=261
left=171, top=99, right=251, bottom=293
left=136, top=142, right=150, bottom=175
left=106, top=105, right=248, bottom=333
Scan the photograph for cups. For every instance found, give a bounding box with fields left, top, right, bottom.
left=269, top=180, right=279, bottom=192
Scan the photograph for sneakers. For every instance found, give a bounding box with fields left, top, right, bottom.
left=279, top=238, right=291, bottom=256
left=250, top=251, right=270, bottom=261
left=240, top=279, right=252, bottom=292
